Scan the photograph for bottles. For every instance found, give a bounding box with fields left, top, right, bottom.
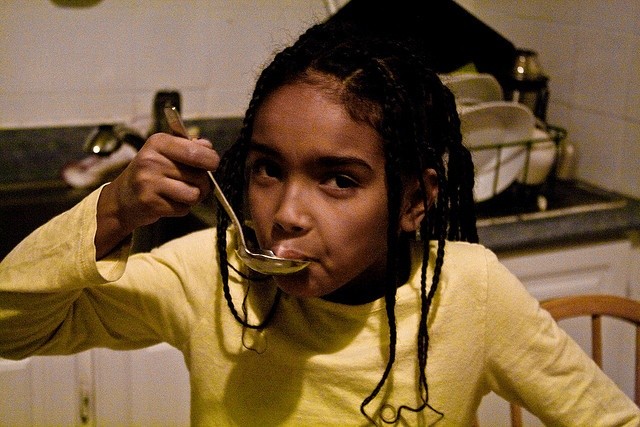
left=128, top=87, right=193, bottom=253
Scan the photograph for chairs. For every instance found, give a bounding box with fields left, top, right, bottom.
left=510, top=295, right=640, bottom=427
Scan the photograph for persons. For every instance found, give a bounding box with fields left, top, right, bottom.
left=0, top=22, right=639, bottom=427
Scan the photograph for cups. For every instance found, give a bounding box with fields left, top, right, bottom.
left=557, top=137, right=584, bottom=179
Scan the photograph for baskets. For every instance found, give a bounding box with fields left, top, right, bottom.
left=460, top=113, right=567, bottom=197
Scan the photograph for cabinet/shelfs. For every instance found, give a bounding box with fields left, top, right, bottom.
left=477, top=239, right=631, bottom=427
left=0, top=343, right=194, bottom=427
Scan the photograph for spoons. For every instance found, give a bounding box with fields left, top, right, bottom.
left=163, top=104, right=310, bottom=273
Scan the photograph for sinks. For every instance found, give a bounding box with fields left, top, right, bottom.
left=1, top=182, right=213, bottom=271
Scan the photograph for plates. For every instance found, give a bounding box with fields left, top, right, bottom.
left=452, top=102, right=535, bottom=203
left=440, top=73, right=503, bottom=108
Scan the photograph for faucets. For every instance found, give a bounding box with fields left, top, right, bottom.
left=84, top=91, right=180, bottom=156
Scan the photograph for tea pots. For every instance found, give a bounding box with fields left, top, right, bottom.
left=512, top=49, right=546, bottom=113
